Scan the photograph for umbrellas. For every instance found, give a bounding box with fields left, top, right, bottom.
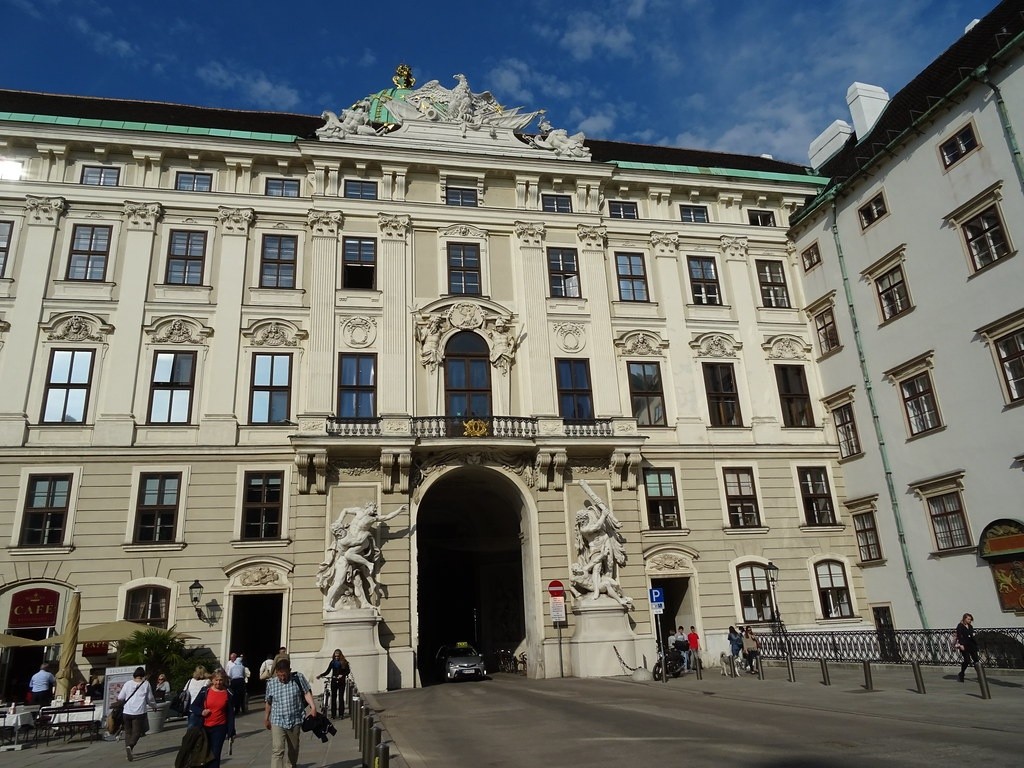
left=55, top=585, right=81, bottom=701
left=0, top=620, right=201, bottom=666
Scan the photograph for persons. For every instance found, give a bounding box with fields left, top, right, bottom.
left=46, top=317, right=804, bottom=359
left=70, top=681, right=86, bottom=698
left=265, top=659, right=316, bottom=768
left=118, top=667, right=159, bottom=761
left=316, top=502, right=409, bottom=612
left=155, top=673, right=170, bottom=693
left=570, top=498, right=633, bottom=609
left=259, top=647, right=290, bottom=682
left=414, top=314, right=445, bottom=374
left=29, top=663, right=55, bottom=715
left=481, top=314, right=515, bottom=377
left=183, top=665, right=236, bottom=768
left=316, top=649, right=350, bottom=719
left=225, top=653, right=251, bottom=715
left=728, top=625, right=760, bottom=675
left=957, top=614, right=979, bottom=681
left=668, top=625, right=700, bottom=673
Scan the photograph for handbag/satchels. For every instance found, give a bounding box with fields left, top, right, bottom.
left=340, top=664, right=351, bottom=677
left=105, top=706, right=123, bottom=734
left=169, top=689, right=191, bottom=714
left=25, top=690, right=33, bottom=704
left=673, top=640, right=690, bottom=651
left=126, top=746, right=133, bottom=761
left=154, top=690, right=166, bottom=702
left=187, top=712, right=203, bottom=728
left=737, top=639, right=743, bottom=648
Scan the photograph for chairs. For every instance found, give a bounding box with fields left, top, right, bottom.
left=0, top=705, right=101, bottom=749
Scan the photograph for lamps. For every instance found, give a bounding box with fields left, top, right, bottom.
left=188, top=580, right=204, bottom=621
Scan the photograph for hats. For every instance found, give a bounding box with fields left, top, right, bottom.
left=280, top=647, right=286, bottom=649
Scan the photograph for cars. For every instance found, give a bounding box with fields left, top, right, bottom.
left=436, top=641, right=487, bottom=683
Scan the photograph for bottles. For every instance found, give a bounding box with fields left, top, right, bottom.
left=10, top=702, right=16, bottom=714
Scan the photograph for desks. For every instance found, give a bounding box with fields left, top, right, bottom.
left=38, top=705, right=103, bottom=741
left=50, top=698, right=103, bottom=706
left=0, top=709, right=35, bottom=750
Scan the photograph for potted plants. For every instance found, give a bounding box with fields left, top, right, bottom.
left=116, top=623, right=185, bottom=735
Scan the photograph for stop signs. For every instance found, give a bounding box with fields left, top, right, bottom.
left=548, top=580, right=564, bottom=597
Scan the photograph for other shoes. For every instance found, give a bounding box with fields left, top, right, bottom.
left=338, top=715, right=344, bottom=720
left=331, top=715, right=336, bottom=719
left=958, top=672, right=966, bottom=683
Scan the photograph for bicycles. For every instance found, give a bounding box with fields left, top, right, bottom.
left=495, top=649, right=528, bottom=676
left=318, top=676, right=340, bottom=719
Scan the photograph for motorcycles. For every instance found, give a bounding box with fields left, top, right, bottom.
left=652, top=640, right=690, bottom=681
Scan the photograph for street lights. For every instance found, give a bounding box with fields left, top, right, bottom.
left=764, top=561, right=779, bottom=633
